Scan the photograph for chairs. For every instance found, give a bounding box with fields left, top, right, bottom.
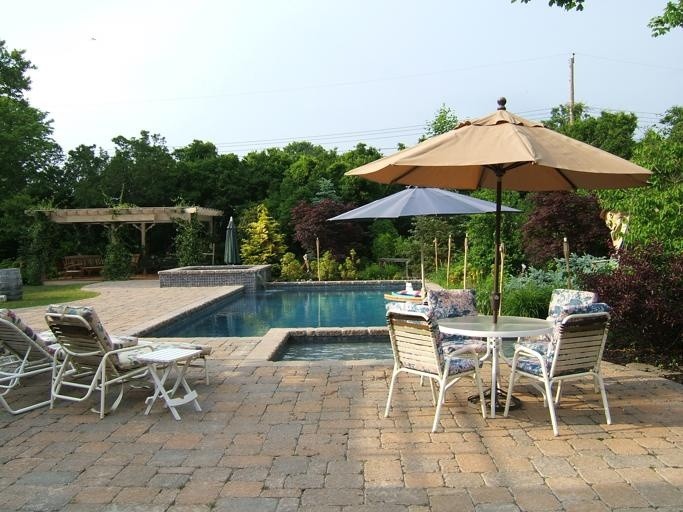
left=515, top=289, right=599, bottom=393
left=504, top=303, right=613, bottom=436
left=0, top=295, right=139, bottom=415
left=44, top=305, right=213, bottom=420
left=384, top=302, right=486, bottom=433
left=420, top=288, right=493, bottom=386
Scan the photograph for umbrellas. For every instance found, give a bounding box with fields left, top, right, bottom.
left=343, top=95, right=655, bottom=323
left=223, top=215, right=241, bottom=266
left=328, top=186, right=525, bottom=291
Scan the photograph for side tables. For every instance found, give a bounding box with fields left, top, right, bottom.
left=137, top=347, right=203, bottom=421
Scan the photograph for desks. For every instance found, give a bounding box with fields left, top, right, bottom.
left=436, top=315, right=553, bottom=412
